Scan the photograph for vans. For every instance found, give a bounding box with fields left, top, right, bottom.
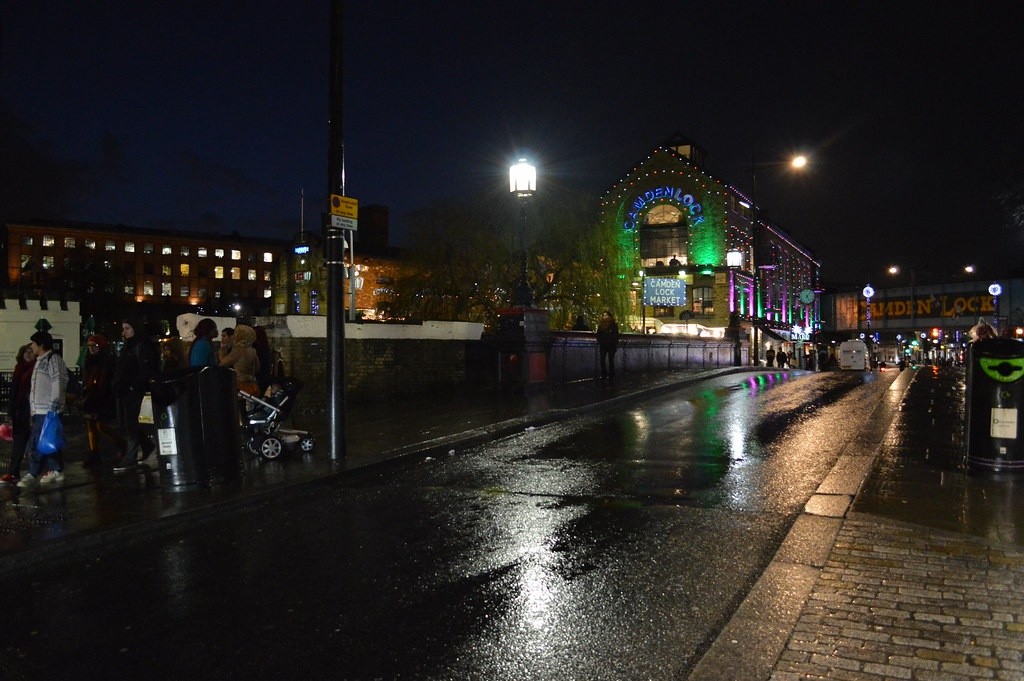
left=840, top=340, right=871, bottom=372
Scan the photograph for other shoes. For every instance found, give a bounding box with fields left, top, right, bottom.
left=139, top=439, right=156, bottom=461
left=112, top=437, right=127, bottom=457
left=82, top=450, right=101, bottom=464
left=113, top=455, right=139, bottom=470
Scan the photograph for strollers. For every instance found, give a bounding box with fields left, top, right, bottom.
left=236, top=375, right=315, bottom=459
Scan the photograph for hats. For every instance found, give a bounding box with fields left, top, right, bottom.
left=122, top=314, right=144, bottom=335
left=87, top=334, right=108, bottom=347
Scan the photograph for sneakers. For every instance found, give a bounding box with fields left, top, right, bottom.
left=39, top=470, right=64, bottom=482
left=16, top=473, right=35, bottom=487
left=0, top=473, right=17, bottom=483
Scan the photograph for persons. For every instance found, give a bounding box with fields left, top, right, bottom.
left=597, top=311, right=619, bottom=383
left=766, top=345, right=796, bottom=369
left=669, top=255, right=681, bottom=266
left=0, top=315, right=285, bottom=487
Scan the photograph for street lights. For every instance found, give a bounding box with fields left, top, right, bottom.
left=863, top=287, right=874, bottom=332
left=988, top=283, right=1001, bottom=331
left=509, top=158, right=537, bottom=309
left=727, top=248, right=743, bottom=328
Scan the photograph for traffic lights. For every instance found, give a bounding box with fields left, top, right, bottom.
left=931, top=329, right=939, bottom=344
